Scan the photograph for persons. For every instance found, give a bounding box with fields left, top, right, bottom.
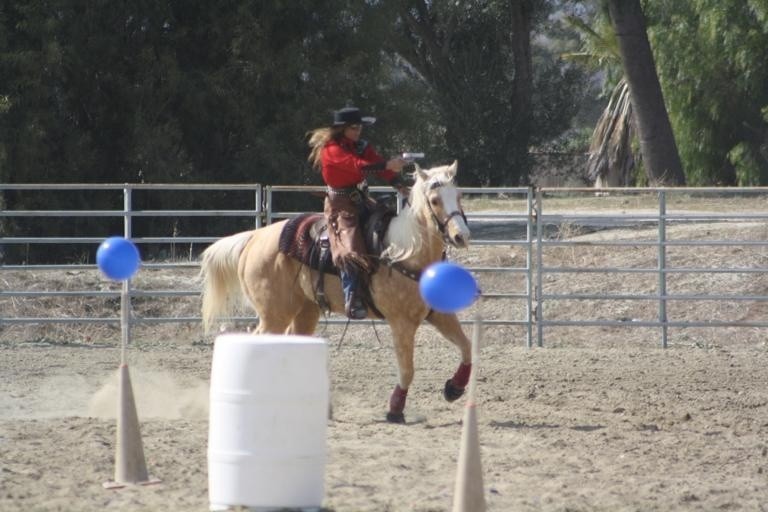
left=308, top=107, right=414, bottom=320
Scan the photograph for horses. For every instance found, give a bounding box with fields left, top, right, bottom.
left=198, top=159, right=474, bottom=425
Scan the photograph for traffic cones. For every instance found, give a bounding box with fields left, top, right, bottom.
left=103, top=362, right=160, bottom=488
left=451, top=404, right=489, bottom=511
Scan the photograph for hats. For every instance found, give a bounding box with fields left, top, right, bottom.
left=323, top=107, right=376, bottom=127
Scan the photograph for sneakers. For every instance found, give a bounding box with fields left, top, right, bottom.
left=350, top=299, right=368, bottom=317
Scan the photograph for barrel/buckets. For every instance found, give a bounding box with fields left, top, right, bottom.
left=207, top=334, right=327, bottom=512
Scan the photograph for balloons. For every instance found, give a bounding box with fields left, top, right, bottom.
left=96, top=236, right=140, bottom=281
left=419, top=261, right=478, bottom=312
